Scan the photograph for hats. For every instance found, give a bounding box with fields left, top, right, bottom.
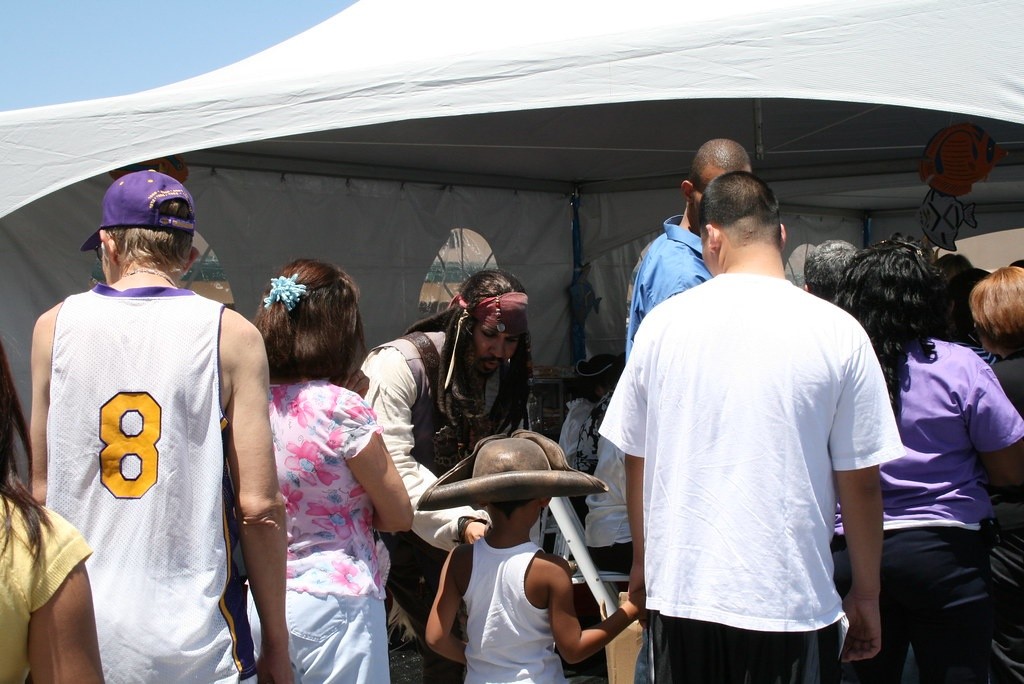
left=80, top=169, right=195, bottom=251
left=574, top=353, right=621, bottom=378
left=417, top=426, right=610, bottom=512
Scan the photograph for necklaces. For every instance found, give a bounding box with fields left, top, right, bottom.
left=122, top=268, right=176, bottom=287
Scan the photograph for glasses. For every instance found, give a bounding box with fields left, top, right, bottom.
left=94, top=243, right=102, bottom=262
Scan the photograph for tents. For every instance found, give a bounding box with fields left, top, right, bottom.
left=0, top=0, right=1024, bottom=488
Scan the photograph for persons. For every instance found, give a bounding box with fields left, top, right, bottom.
left=425, top=438, right=647, bottom=684
left=236, top=257, right=414, bottom=684
left=559, top=354, right=633, bottom=576
left=30, top=169, right=295, bottom=684
left=803, top=232, right=1024, bottom=684
left=598, top=140, right=907, bottom=684
left=359, top=270, right=532, bottom=684
left=0, top=342, right=106, bottom=684
